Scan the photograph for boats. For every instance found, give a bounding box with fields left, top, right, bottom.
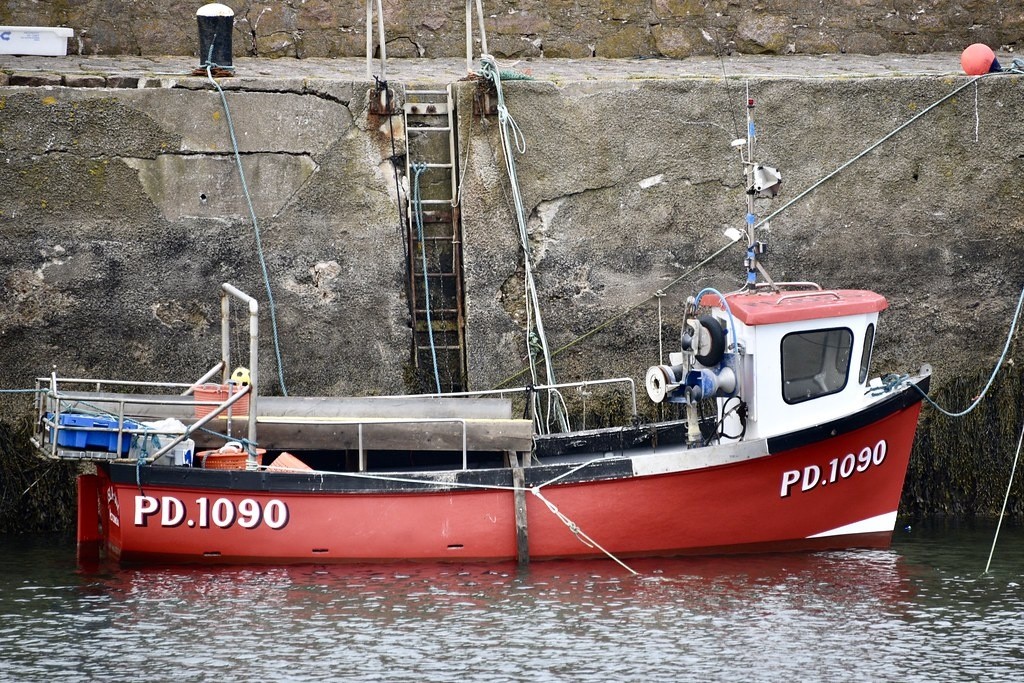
left=32, top=71, right=934, bottom=568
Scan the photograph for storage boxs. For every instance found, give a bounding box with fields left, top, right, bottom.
left=47, top=412, right=138, bottom=453
left=0, top=25, right=74, bottom=56
left=129, top=433, right=195, bottom=466
